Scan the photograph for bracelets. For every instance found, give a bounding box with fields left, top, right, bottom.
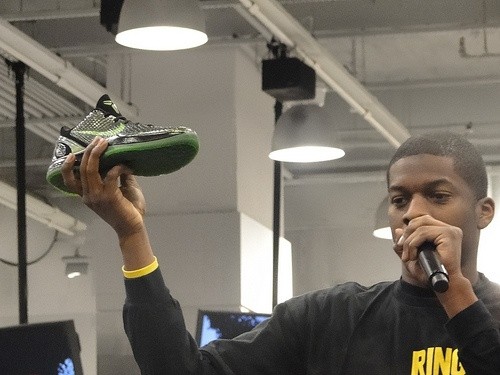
left=121, top=256, right=158, bottom=279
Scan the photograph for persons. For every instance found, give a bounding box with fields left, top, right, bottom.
left=60, top=130, right=500, bottom=375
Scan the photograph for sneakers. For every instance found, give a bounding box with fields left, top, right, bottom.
left=46, top=95, right=198, bottom=194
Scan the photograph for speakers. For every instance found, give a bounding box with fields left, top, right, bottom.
left=261, top=57, right=316, bottom=102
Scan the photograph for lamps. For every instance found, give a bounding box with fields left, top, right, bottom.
left=268, top=105, right=346, bottom=163
left=115, top=0, right=209, bottom=52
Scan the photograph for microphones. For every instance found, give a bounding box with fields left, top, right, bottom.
left=417, top=242, right=449, bottom=293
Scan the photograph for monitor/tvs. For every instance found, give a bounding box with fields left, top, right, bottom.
left=197, top=310, right=272, bottom=348
left=0, top=320, right=83, bottom=375
